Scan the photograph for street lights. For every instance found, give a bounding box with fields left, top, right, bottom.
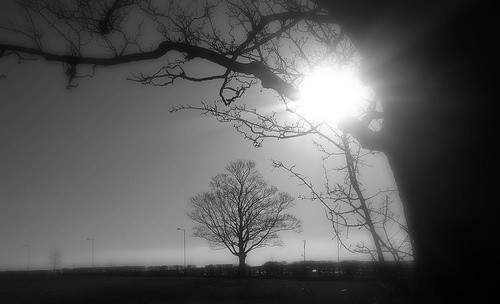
left=87, top=238, right=94, bottom=267
left=23, top=245, right=29, bottom=271
left=177, top=228, right=186, bottom=266
